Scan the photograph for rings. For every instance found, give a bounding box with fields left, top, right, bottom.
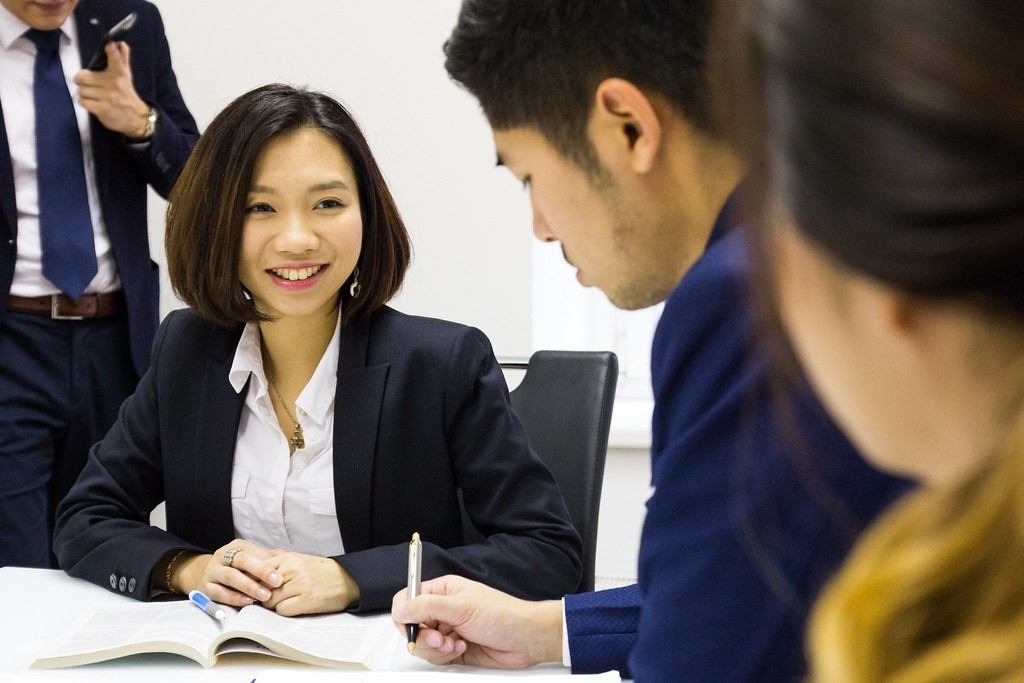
left=225, top=548, right=243, bottom=567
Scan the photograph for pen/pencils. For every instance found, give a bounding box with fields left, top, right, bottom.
left=188, top=589, right=228, bottom=625
left=401, top=529, right=424, bottom=654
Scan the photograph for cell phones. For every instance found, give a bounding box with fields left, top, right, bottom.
left=88, top=12, right=136, bottom=71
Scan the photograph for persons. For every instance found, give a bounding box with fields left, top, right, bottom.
left=394, top=1, right=920, bottom=681
left=58, top=85, right=587, bottom=620
left=704, top=0, right=1024, bottom=682
left=0, top=0, right=203, bottom=567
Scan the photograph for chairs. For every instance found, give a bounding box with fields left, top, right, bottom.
left=498, top=350, right=619, bottom=594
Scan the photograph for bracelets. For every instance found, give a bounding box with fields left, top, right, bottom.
left=142, top=108, right=161, bottom=135
left=165, top=551, right=187, bottom=595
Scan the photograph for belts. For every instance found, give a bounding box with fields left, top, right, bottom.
left=2, top=288, right=126, bottom=320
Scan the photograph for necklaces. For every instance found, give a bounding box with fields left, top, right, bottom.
left=268, top=366, right=314, bottom=451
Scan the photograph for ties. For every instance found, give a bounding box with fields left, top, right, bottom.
left=21, top=26, right=99, bottom=303
